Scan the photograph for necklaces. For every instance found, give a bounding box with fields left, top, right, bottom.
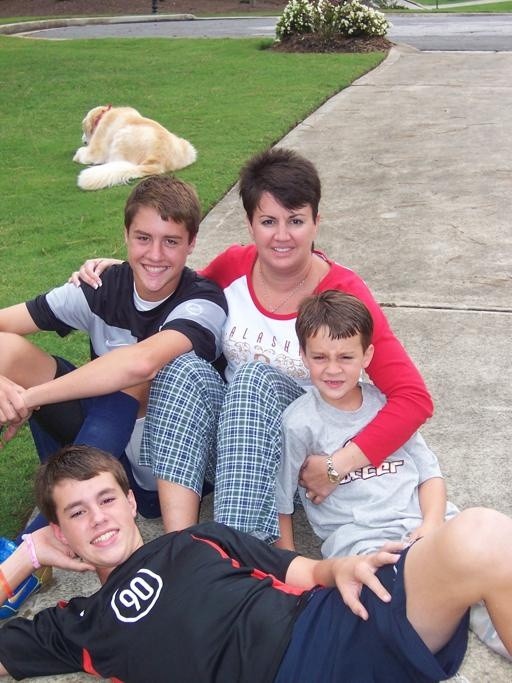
left=257, top=265, right=311, bottom=314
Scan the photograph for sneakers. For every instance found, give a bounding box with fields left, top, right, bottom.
left=0, top=535, right=53, bottom=619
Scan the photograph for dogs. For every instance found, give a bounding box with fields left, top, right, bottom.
left=72, top=104, right=197, bottom=191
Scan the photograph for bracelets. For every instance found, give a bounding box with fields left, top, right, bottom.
left=327, top=456, right=340, bottom=484
left=0, top=567, right=14, bottom=596
left=26, top=534, right=42, bottom=569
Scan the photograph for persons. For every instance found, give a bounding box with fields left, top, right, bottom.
left=67, top=152, right=435, bottom=544
left=0, top=173, right=228, bottom=623
left=0, top=444, right=512, bottom=682
left=275, top=288, right=512, bottom=661
